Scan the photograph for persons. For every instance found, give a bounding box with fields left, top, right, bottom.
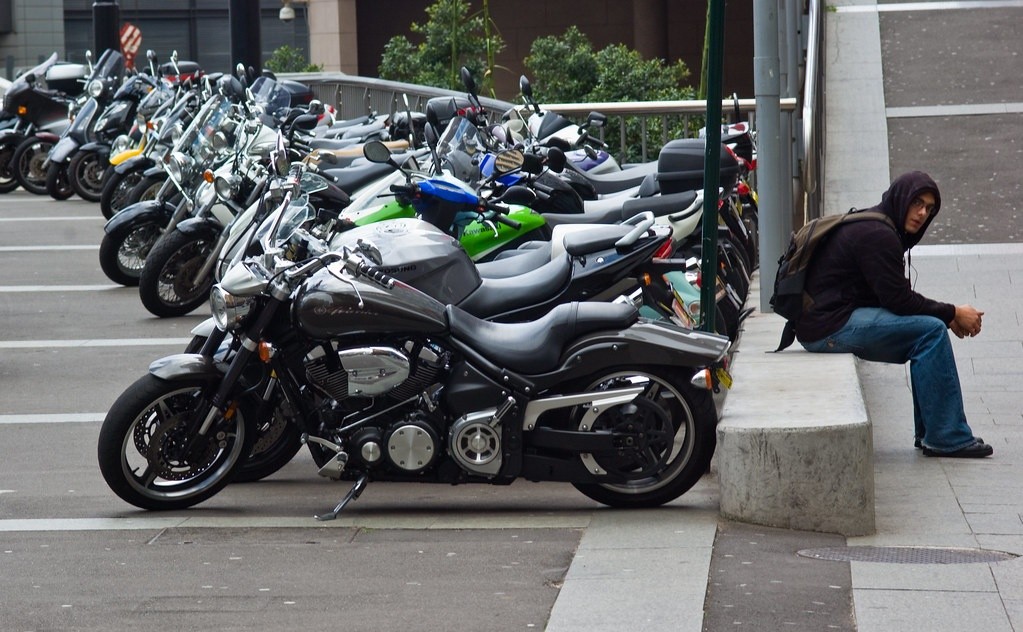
left=775, top=171, right=996, bottom=460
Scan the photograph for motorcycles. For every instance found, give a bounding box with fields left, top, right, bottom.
left=0, top=48, right=762, bottom=484
left=97, top=191, right=735, bottom=522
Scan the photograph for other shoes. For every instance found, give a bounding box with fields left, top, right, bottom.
left=923, top=444, right=993, bottom=457
left=914, top=437, right=984, bottom=449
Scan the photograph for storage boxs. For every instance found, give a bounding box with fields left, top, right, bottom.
left=698, top=122, right=753, bottom=171
left=426, top=96, right=476, bottom=136
left=46, top=64, right=90, bottom=96
left=162, top=61, right=205, bottom=86
left=274, top=80, right=313, bottom=108
left=658, top=137, right=738, bottom=200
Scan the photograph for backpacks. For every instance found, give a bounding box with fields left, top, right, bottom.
left=768, top=207, right=903, bottom=323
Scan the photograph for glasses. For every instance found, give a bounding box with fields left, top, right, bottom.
left=911, top=198, right=936, bottom=216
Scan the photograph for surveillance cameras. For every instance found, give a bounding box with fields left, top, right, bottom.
left=279, top=0, right=296, bottom=25
